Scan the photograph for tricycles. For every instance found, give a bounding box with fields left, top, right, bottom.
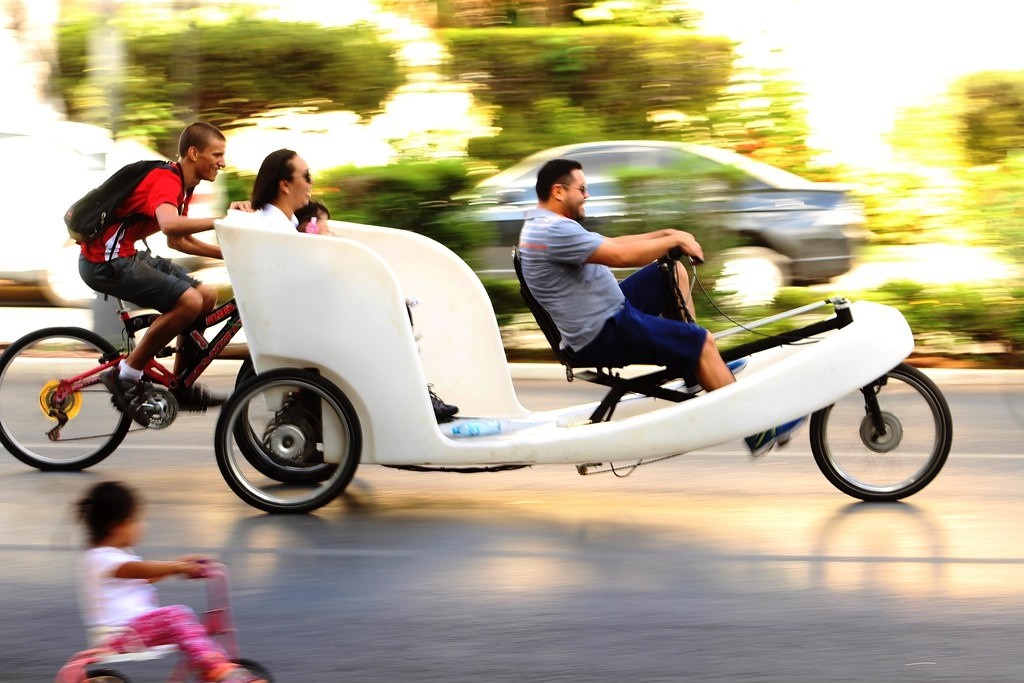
left=211, top=209, right=954, bottom=514
left=54, top=559, right=278, bottom=683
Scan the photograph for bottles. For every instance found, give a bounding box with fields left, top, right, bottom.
left=438, top=416, right=532, bottom=438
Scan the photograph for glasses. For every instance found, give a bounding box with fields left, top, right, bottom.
left=558, top=183, right=586, bottom=195
left=287, top=169, right=311, bottom=183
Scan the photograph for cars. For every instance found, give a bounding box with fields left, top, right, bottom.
left=471, top=136, right=873, bottom=310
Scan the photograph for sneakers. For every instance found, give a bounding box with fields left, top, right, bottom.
left=99, top=365, right=150, bottom=428
left=744, top=413, right=810, bottom=457
left=683, top=358, right=748, bottom=394
left=168, top=381, right=228, bottom=409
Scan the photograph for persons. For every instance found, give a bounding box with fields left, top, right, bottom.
left=252, top=148, right=459, bottom=422
left=78, top=122, right=254, bottom=404
left=520, top=159, right=809, bottom=454
left=76, top=481, right=268, bottom=683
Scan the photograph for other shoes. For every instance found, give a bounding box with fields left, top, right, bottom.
left=427, top=383, right=459, bottom=419
left=204, top=662, right=250, bottom=680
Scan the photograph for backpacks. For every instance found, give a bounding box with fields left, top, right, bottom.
left=64, top=159, right=194, bottom=245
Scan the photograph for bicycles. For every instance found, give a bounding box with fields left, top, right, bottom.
left=0, top=290, right=244, bottom=474
